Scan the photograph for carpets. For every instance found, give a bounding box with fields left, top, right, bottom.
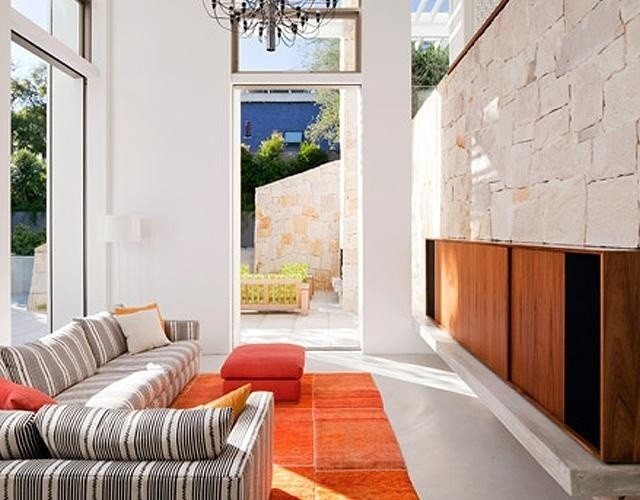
left=167, top=373, right=419, bottom=499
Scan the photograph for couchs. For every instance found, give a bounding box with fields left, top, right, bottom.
left=0, top=303, right=274, bottom=500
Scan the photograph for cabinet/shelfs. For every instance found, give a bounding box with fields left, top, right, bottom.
left=425, top=239, right=639, bottom=464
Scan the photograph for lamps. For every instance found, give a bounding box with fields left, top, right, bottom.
left=104, top=214, right=142, bottom=307
left=202, top=0, right=339, bottom=51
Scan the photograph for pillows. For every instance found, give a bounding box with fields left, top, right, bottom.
left=0, top=376, right=58, bottom=410
left=37, top=403, right=232, bottom=461
left=206, top=383, right=251, bottom=417
left=0, top=412, right=47, bottom=459
left=115, top=308, right=170, bottom=355
left=1, top=322, right=97, bottom=397
left=115, top=303, right=165, bottom=329
left=73, top=312, right=127, bottom=366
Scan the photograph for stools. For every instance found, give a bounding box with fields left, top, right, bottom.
left=221, top=343, right=304, bottom=401
